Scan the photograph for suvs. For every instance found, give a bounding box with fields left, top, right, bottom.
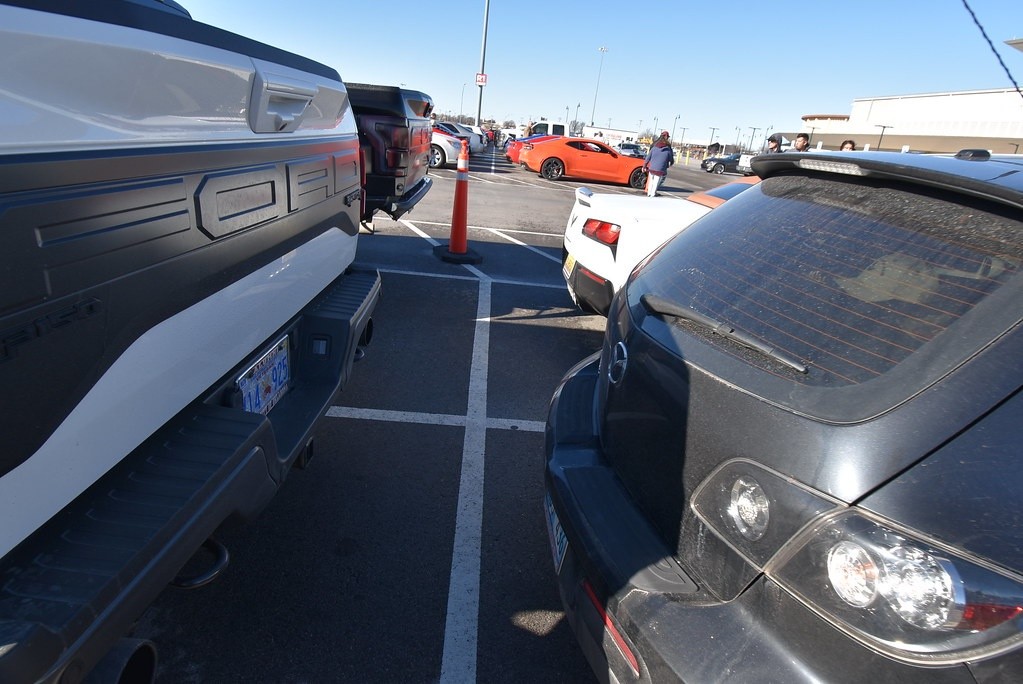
left=538, top=146, right=1023, bottom=682
left=3, top=0, right=387, bottom=677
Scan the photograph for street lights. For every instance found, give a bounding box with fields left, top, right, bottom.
left=709, top=127, right=719, bottom=145
left=591, top=46, right=609, bottom=128
left=748, top=126, right=761, bottom=152
left=654, top=116, right=658, bottom=138
left=670, top=115, right=680, bottom=148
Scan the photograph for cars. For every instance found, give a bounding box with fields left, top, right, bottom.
left=701, top=152, right=745, bottom=176
left=561, top=176, right=788, bottom=324
left=519, top=138, right=667, bottom=189
left=430, top=121, right=527, bottom=170
left=613, top=142, right=648, bottom=160
left=506, top=135, right=598, bottom=165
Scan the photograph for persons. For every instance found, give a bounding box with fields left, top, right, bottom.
left=523, top=121, right=534, bottom=138
left=430, top=113, right=437, bottom=126
left=785, top=133, right=811, bottom=153
left=760, top=133, right=784, bottom=155
left=479, top=128, right=498, bottom=148
left=841, top=140, right=856, bottom=152
left=640, top=131, right=674, bottom=197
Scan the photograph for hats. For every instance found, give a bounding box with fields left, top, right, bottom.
left=766, top=135, right=775, bottom=141
left=661, top=131, right=671, bottom=137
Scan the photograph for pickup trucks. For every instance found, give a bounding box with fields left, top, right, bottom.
left=344, top=83, right=436, bottom=223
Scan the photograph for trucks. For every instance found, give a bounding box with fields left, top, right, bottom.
left=525, top=121, right=610, bottom=150
